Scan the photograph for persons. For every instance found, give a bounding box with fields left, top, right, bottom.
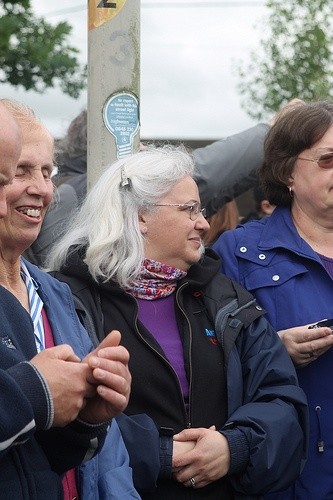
left=0, top=98, right=333, bottom=500
left=47, top=144, right=308, bottom=500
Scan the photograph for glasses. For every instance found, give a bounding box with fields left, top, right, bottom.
left=150, top=201, right=207, bottom=222
left=295, top=152, right=333, bottom=169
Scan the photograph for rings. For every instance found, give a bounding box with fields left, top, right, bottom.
left=190, top=477, right=196, bottom=487
left=122, top=386, right=129, bottom=395
